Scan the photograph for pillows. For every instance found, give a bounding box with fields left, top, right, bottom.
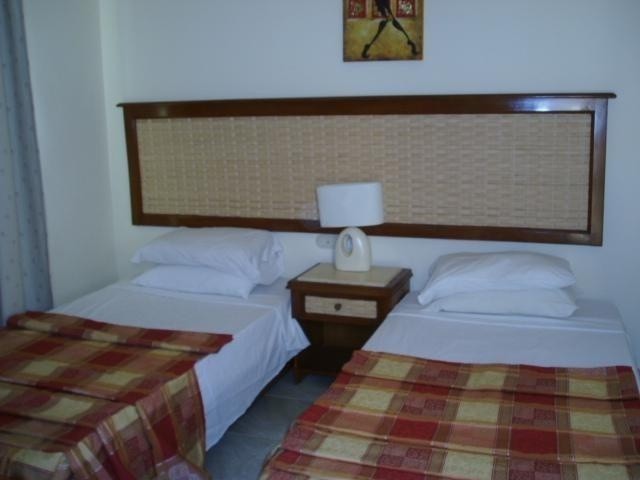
left=423, top=294, right=577, bottom=318
left=132, top=227, right=285, bottom=274
left=418, top=251, right=576, bottom=305
left=132, top=264, right=256, bottom=298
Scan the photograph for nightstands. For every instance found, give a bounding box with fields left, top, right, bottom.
left=287, top=261, right=413, bottom=384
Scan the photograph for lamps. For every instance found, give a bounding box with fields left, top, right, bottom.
left=314, top=181, right=384, bottom=270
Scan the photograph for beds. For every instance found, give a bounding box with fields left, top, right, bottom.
left=1, top=273, right=311, bottom=478
left=264, top=290, right=640, bottom=479
